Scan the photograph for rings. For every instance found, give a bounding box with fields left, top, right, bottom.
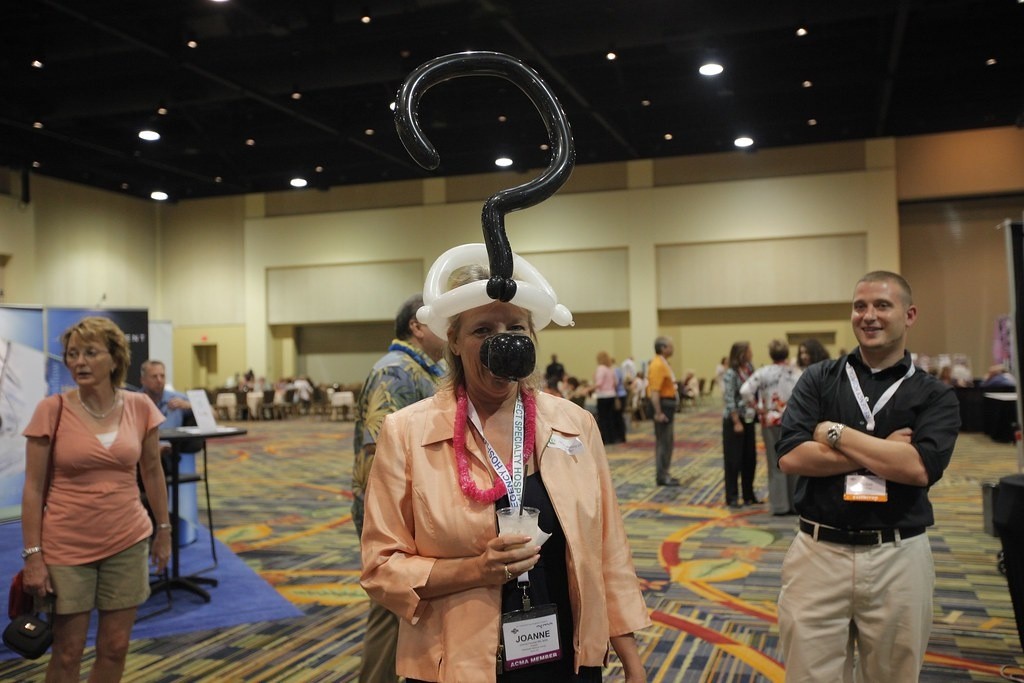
left=504, top=565, right=512, bottom=579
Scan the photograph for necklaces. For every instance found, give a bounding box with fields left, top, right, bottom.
left=454, top=381, right=536, bottom=504
left=77, top=385, right=117, bottom=418
left=390, top=344, right=431, bottom=375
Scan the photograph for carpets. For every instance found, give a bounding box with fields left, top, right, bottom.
left=0, top=514, right=303, bottom=659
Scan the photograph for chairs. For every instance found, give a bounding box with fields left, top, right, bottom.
left=629, top=378, right=720, bottom=419
left=213, top=385, right=363, bottom=422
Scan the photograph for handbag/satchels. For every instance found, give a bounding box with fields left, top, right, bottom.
left=8, top=570, right=32, bottom=622
left=3, top=614, right=53, bottom=660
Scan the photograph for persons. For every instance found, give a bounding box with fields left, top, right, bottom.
left=774, top=271, right=960, bottom=683
left=352, top=291, right=449, bottom=683
left=646, top=336, right=681, bottom=485
left=722, top=340, right=764, bottom=506
left=137, top=360, right=219, bottom=492
left=359, top=263, right=654, bottom=683
left=718, top=356, right=729, bottom=393
left=796, top=337, right=828, bottom=370
left=939, top=363, right=1016, bottom=387
left=740, top=339, right=806, bottom=516
left=226, top=371, right=317, bottom=420
left=21, top=316, right=172, bottom=683
left=545, top=350, right=650, bottom=445
left=681, top=369, right=699, bottom=399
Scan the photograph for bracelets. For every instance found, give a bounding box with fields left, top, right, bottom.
left=157, top=523, right=171, bottom=529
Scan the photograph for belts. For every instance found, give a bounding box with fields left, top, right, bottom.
left=800, top=518, right=926, bottom=545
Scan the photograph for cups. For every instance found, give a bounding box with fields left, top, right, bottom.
left=496, top=507, right=541, bottom=572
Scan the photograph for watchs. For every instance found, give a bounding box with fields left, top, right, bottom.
left=826, top=424, right=845, bottom=448
left=22, top=546, right=41, bottom=559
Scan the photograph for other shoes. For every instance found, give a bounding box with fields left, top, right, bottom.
left=744, top=497, right=764, bottom=506
left=728, top=500, right=741, bottom=508
left=657, top=478, right=679, bottom=486
left=774, top=510, right=798, bottom=516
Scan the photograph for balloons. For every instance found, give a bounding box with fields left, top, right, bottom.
left=394, top=48, right=575, bottom=337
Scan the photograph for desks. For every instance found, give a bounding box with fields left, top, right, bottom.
left=148, top=425, right=247, bottom=602
left=218, top=389, right=284, bottom=421
